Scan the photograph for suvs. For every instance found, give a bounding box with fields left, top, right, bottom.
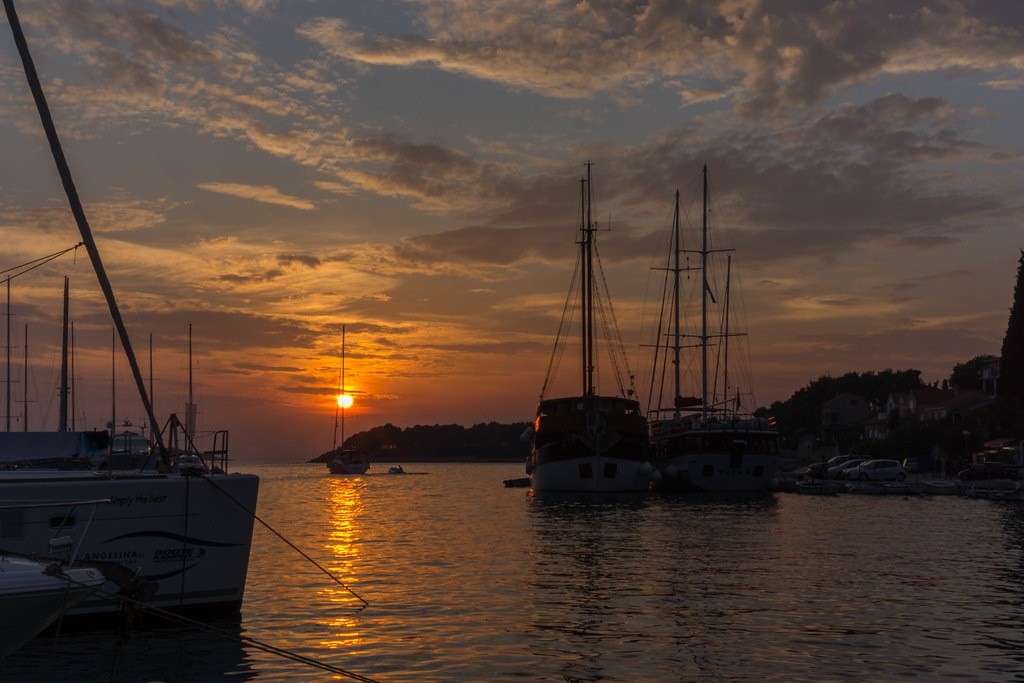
left=806, top=453, right=873, bottom=480
left=842, top=459, right=908, bottom=482
left=825, top=458, right=867, bottom=478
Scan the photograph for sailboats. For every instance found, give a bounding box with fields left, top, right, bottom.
left=635, top=164, right=781, bottom=500
left=0, top=0, right=261, bottom=620
left=916, top=456, right=965, bottom=496
left=326, top=325, right=371, bottom=474
left=795, top=459, right=839, bottom=494
left=843, top=462, right=880, bottom=494
left=517, top=159, right=662, bottom=502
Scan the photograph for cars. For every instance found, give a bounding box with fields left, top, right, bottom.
left=903, top=457, right=924, bottom=473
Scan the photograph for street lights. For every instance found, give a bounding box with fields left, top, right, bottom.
left=962, top=430, right=971, bottom=456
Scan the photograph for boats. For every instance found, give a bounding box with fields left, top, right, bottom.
left=875, top=476, right=913, bottom=494
left=504, top=475, right=530, bottom=488
left=0, top=497, right=142, bottom=660
left=388, top=465, right=407, bottom=474
